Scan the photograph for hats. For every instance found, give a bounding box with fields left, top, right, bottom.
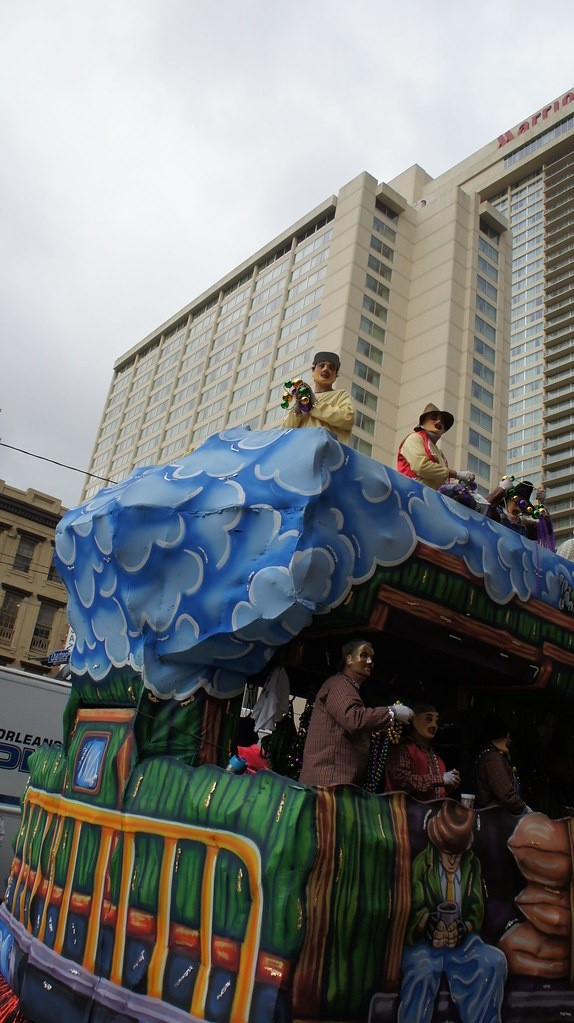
left=313, top=352, right=340, bottom=370
left=507, top=481, right=535, bottom=516
left=414, top=403, right=454, bottom=433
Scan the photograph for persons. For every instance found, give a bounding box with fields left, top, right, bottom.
left=485, top=478, right=552, bottom=542
left=299, top=638, right=414, bottom=787
left=397, top=403, right=475, bottom=491
left=283, top=351, right=354, bottom=445
left=474, top=721, right=534, bottom=814
left=385, top=703, right=461, bottom=800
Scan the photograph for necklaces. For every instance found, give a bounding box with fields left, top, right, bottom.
left=281, top=379, right=311, bottom=412
left=502, top=476, right=547, bottom=519
left=365, top=701, right=402, bottom=791
left=287, top=698, right=311, bottom=767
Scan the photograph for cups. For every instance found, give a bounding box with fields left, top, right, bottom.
left=225, top=755, right=248, bottom=776
left=460, top=794, right=475, bottom=809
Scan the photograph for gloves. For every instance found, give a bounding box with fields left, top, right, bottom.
left=392, top=705, right=416, bottom=724
left=499, top=479, right=513, bottom=493
left=443, top=769, right=461, bottom=785
left=456, top=470, right=475, bottom=484
